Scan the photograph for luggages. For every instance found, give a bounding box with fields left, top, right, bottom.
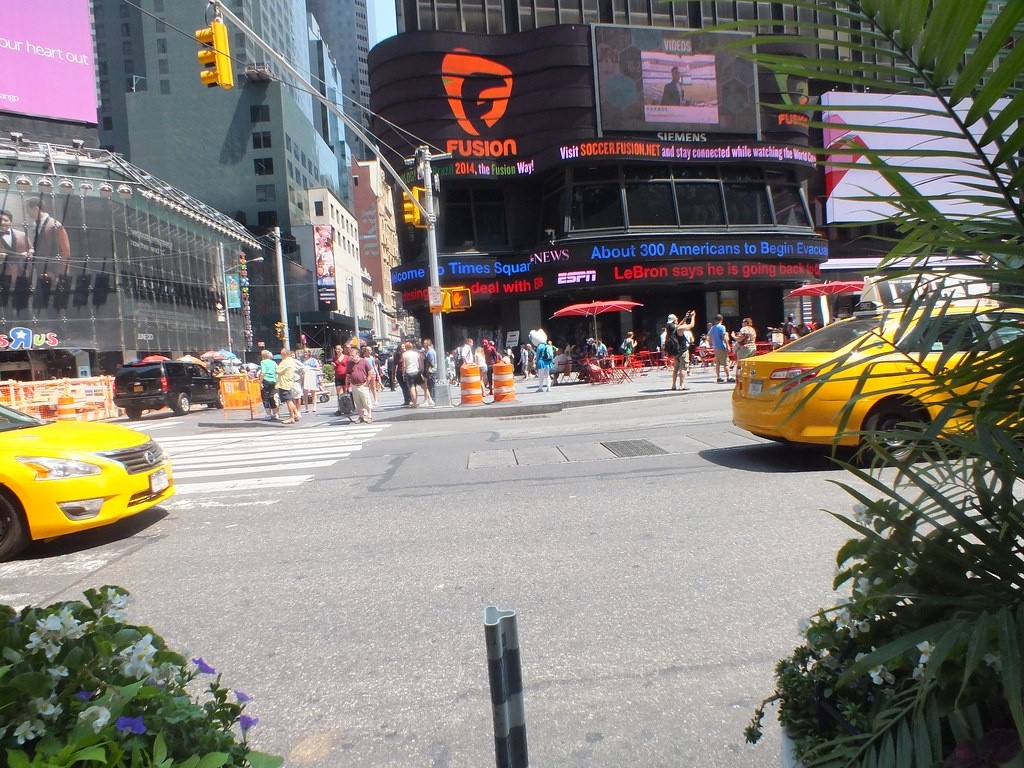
left=339, top=392, right=354, bottom=415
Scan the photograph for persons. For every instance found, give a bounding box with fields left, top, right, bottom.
left=25, top=196, right=71, bottom=286
left=621, top=332, right=638, bottom=374
left=660, top=310, right=696, bottom=391
left=259, top=333, right=514, bottom=426
left=49, top=375, right=115, bottom=415
left=784, top=312, right=835, bottom=346
left=662, top=67, right=688, bottom=105
left=518, top=340, right=615, bottom=394
left=210, top=362, right=258, bottom=380
left=0, top=209, right=35, bottom=289
left=699, top=312, right=757, bottom=382
left=315, top=226, right=335, bottom=287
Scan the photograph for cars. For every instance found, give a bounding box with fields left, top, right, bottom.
left=731, top=274, right=1024, bottom=468
left=0, top=402, right=178, bottom=564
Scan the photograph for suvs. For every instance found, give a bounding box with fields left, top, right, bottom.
left=113, top=360, right=224, bottom=420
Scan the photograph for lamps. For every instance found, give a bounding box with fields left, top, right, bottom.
left=72, top=139, right=85, bottom=154
left=544, top=229, right=556, bottom=241
left=78, top=180, right=95, bottom=193
left=98, top=181, right=115, bottom=197
left=10, top=132, right=23, bottom=145
left=116, top=183, right=134, bottom=199
left=14, top=174, right=33, bottom=190
left=135, top=187, right=262, bottom=252
left=36, top=175, right=54, bottom=192
left=0, top=173, right=11, bottom=188
left=57, top=177, right=75, bottom=195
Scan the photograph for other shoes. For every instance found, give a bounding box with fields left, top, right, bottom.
left=272, top=414, right=279, bottom=420
left=727, top=378, right=735, bottom=382
left=295, top=416, right=300, bottom=422
left=525, top=377, right=529, bottom=381
left=536, top=389, right=544, bottom=392
left=553, top=382, right=557, bottom=386
left=282, top=417, right=294, bottom=424
left=263, top=416, right=271, bottom=421
left=488, top=392, right=493, bottom=395
left=367, top=418, right=371, bottom=424
left=402, top=400, right=435, bottom=409
left=355, top=417, right=363, bottom=423
left=482, top=394, right=486, bottom=397
left=672, top=386, right=676, bottom=390
left=717, top=379, right=725, bottom=383
left=546, top=389, right=550, bottom=392
left=679, top=386, right=689, bottom=390
left=312, top=406, right=316, bottom=412
left=305, top=409, right=309, bottom=413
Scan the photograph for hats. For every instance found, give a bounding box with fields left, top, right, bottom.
left=667, top=314, right=675, bottom=324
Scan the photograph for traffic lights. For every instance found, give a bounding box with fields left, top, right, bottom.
left=403, top=187, right=420, bottom=225
left=451, top=289, right=472, bottom=309
left=194, top=21, right=234, bottom=89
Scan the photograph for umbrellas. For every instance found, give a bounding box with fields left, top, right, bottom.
left=247, top=362, right=259, bottom=369
left=783, top=275, right=864, bottom=323
left=202, top=347, right=242, bottom=366
left=177, top=353, right=208, bottom=368
left=144, top=354, right=170, bottom=362
left=550, top=297, right=643, bottom=355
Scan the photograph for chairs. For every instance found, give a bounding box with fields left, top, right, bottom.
left=925, top=322, right=985, bottom=350
left=695, top=341, right=775, bottom=369
left=561, top=350, right=672, bottom=386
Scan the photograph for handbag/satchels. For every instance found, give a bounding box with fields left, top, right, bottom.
left=619, top=340, right=626, bottom=354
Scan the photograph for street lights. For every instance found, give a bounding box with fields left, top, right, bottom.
left=219, top=242, right=264, bottom=354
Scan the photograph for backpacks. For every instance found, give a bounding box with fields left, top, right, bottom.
left=665, top=326, right=681, bottom=355
